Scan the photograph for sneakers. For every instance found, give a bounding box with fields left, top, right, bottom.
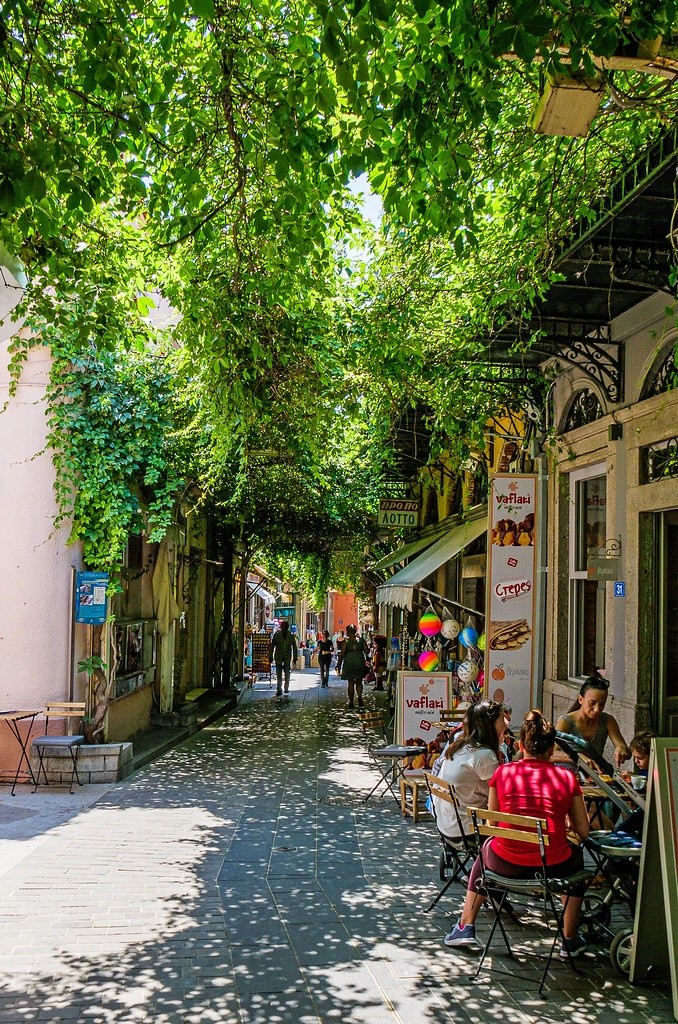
left=445, top=918, right=477, bottom=945
left=559, top=933, right=586, bottom=957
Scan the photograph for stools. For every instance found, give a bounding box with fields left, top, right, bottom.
left=400, top=778, right=432, bottom=823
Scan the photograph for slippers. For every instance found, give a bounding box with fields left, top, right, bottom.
left=585, top=874, right=610, bottom=889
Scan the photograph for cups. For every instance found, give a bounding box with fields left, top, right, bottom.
left=630, top=775, right=646, bottom=790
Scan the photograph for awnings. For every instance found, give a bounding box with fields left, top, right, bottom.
left=375, top=516, right=487, bottom=619
left=361, top=529, right=448, bottom=587
left=263, top=584, right=292, bottom=602
left=247, top=583, right=276, bottom=617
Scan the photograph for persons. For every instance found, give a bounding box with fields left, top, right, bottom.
left=311, top=630, right=334, bottom=688
left=268, top=622, right=297, bottom=697
left=335, top=624, right=370, bottom=708
left=372, top=636, right=387, bottom=690
left=445, top=710, right=589, bottom=958
left=554, top=677, right=659, bottom=889
left=431, top=700, right=523, bottom=914
left=332, top=629, right=346, bottom=677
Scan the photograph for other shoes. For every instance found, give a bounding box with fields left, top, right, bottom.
left=358, top=697, right=364, bottom=707
left=460, top=869, right=472, bottom=884
left=348, top=702, right=354, bottom=708
left=321, top=684, right=328, bottom=688
left=276, top=692, right=282, bottom=696
left=284, top=691, right=289, bottom=693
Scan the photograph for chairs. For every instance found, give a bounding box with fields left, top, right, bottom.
left=357, top=711, right=427, bottom=809
left=422, top=771, right=584, bottom=1000
left=30, top=702, right=86, bottom=794
left=438, top=709, right=468, bottom=731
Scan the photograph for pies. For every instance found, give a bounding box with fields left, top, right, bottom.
left=489, top=619, right=532, bottom=650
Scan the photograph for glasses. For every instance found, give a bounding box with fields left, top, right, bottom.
left=584, top=677, right=609, bottom=689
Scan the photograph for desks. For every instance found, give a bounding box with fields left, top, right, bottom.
left=0, top=710, right=43, bottom=796
left=579, top=785, right=644, bottom=831
